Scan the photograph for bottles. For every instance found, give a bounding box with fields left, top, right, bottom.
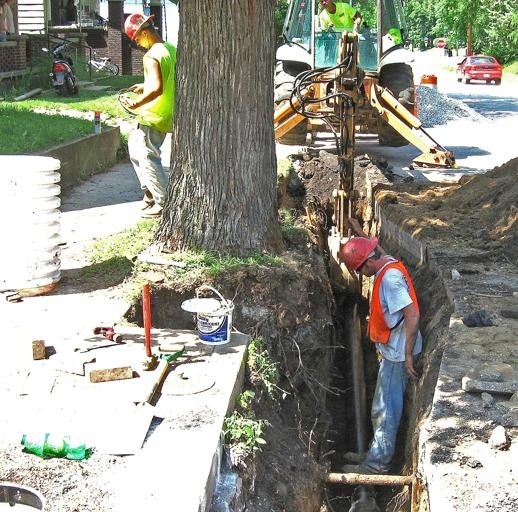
left=95, top=111, right=102, bottom=133
left=451, top=269, right=460, bottom=280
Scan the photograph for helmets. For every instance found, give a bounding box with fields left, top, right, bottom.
left=341, top=238, right=379, bottom=270
left=125, top=14, right=155, bottom=41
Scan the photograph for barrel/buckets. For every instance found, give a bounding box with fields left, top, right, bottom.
left=420, top=74, right=437, bottom=93
left=182, top=285, right=235, bottom=345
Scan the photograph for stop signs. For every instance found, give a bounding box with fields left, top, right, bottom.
left=436, top=40, right=445, bottom=48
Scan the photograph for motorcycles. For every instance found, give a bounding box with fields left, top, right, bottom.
left=40, top=40, right=81, bottom=96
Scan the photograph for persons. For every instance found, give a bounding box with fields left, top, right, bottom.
left=65, top=0, right=79, bottom=25
left=317, top=0, right=368, bottom=67
left=0, top=1, right=16, bottom=42
left=122, top=12, right=179, bottom=220
left=384, top=26, right=404, bottom=46
left=336, top=217, right=426, bottom=474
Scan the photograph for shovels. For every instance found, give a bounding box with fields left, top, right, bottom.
left=105, top=358, right=169, bottom=454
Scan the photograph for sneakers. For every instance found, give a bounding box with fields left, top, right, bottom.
left=343, top=451, right=366, bottom=464
left=143, top=203, right=163, bottom=217
left=342, top=464, right=373, bottom=475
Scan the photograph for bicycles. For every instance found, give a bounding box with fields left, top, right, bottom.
left=86, top=50, right=120, bottom=76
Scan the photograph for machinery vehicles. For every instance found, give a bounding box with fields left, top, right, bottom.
left=272, top=1, right=454, bottom=295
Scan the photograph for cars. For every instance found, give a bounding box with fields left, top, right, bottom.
left=454, top=55, right=503, bottom=86
